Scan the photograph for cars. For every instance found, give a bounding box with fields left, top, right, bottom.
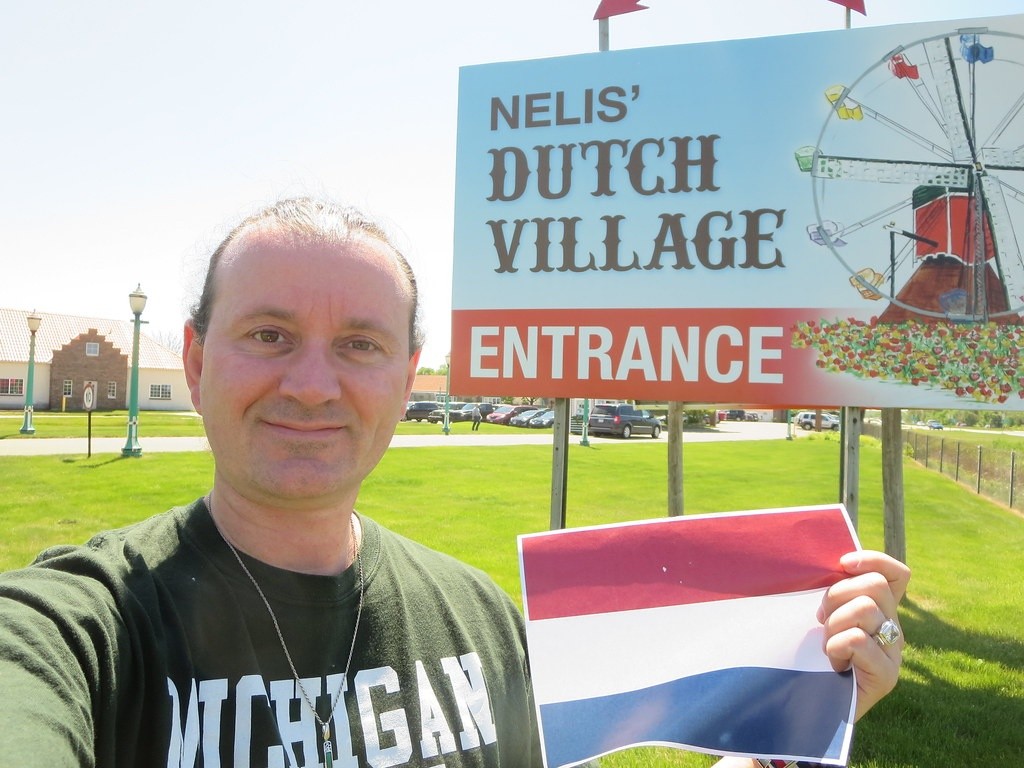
left=928, top=423, right=943, bottom=430
left=509, top=410, right=546, bottom=427
left=716, top=412, right=727, bottom=423
left=530, top=411, right=554, bottom=428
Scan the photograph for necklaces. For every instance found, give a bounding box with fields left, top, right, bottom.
left=208, top=491, right=365, bottom=768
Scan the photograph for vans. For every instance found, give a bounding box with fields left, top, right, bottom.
left=486, top=406, right=539, bottom=425
left=400, top=402, right=438, bottom=422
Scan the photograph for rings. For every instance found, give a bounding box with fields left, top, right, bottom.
left=872, top=619, right=901, bottom=646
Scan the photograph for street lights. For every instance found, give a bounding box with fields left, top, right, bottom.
left=20, top=309, right=42, bottom=434
left=122, top=283, right=150, bottom=457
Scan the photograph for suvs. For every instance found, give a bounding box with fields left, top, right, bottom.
left=726, top=410, right=745, bottom=421
left=588, top=404, right=662, bottom=439
left=451, top=403, right=493, bottom=422
left=428, top=403, right=466, bottom=424
left=797, top=412, right=839, bottom=431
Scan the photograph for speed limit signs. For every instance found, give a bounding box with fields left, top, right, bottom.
left=83, top=385, right=94, bottom=411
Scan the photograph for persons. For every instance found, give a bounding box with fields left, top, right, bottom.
left=1, top=195, right=912, bottom=768
left=472, top=403, right=482, bottom=431
